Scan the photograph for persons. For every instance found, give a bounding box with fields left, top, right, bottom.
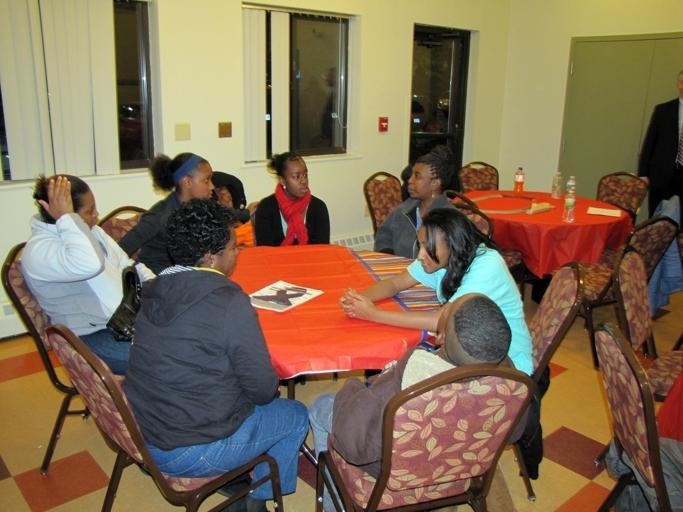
left=121, top=198, right=309, bottom=512
left=320, top=66, right=335, bottom=147
left=340, top=208, right=534, bottom=380
left=211, top=172, right=256, bottom=247
left=251, top=151, right=331, bottom=247
left=309, top=293, right=617, bottom=511
left=638, top=70, right=683, bottom=219
left=118, top=153, right=215, bottom=274
left=21, top=174, right=158, bottom=376
left=372, top=145, right=456, bottom=258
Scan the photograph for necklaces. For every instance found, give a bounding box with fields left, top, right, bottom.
left=420, top=201, right=427, bottom=213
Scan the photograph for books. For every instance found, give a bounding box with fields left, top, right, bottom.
left=247, top=281, right=324, bottom=313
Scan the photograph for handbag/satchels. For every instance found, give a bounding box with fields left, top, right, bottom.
left=107, top=265, right=142, bottom=342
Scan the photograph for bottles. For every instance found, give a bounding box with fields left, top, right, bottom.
left=515, top=167, right=525, bottom=192
left=552, top=171, right=576, bottom=223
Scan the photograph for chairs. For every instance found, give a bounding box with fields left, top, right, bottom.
left=98, top=206, right=148, bottom=262
left=212, top=171, right=246, bottom=210
left=1, top=242, right=123, bottom=475
left=591, top=323, right=670, bottom=510
left=315, top=364, right=533, bottom=510
left=47, top=325, right=284, bottom=512
left=507, top=261, right=583, bottom=502
left=616, top=245, right=682, bottom=401
left=314, top=161, right=683, bottom=371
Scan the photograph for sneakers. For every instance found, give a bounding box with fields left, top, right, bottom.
left=216, top=478, right=249, bottom=498
